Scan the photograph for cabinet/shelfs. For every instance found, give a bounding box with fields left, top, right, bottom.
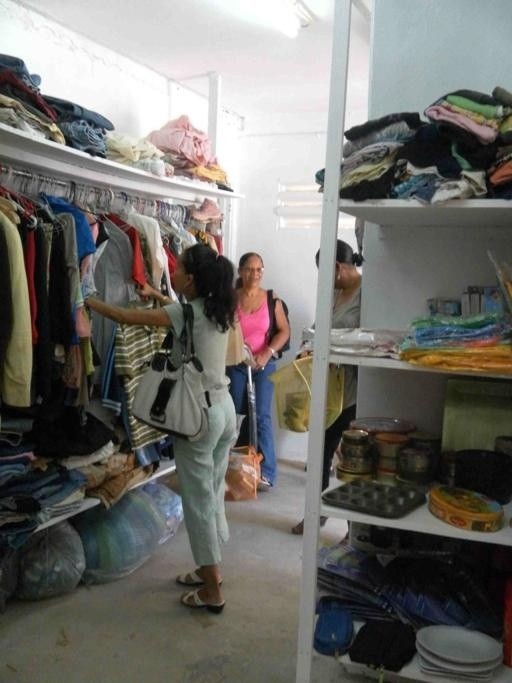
left=310, top=192, right=512, bottom=683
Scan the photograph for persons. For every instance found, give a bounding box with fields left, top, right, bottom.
left=290, top=239, right=363, bottom=550
left=80, top=243, right=244, bottom=614
left=224, top=251, right=292, bottom=491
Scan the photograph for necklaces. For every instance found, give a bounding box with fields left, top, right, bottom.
left=333, top=288, right=357, bottom=313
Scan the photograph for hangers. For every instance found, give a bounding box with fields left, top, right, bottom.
left=0, top=166, right=193, bottom=249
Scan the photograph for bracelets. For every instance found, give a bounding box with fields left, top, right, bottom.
left=157, top=293, right=169, bottom=304
left=266, top=346, right=275, bottom=355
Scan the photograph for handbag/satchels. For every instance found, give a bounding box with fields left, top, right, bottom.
left=129, top=303, right=212, bottom=444
left=268, top=357, right=346, bottom=433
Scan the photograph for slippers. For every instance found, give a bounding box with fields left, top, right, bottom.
left=176, top=570, right=223, bottom=587
left=180, top=587, right=226, bottom=614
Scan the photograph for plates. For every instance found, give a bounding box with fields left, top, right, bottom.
left=414, top=623, right=504, bottom=682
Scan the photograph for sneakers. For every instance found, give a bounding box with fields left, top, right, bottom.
left=257, top=477, right=272, bottom=491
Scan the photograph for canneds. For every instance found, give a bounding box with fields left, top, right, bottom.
left=336, top=414, right=437, bottom=492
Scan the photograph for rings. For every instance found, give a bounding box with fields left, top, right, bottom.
left=261, top=367, right=265, bottom=371
